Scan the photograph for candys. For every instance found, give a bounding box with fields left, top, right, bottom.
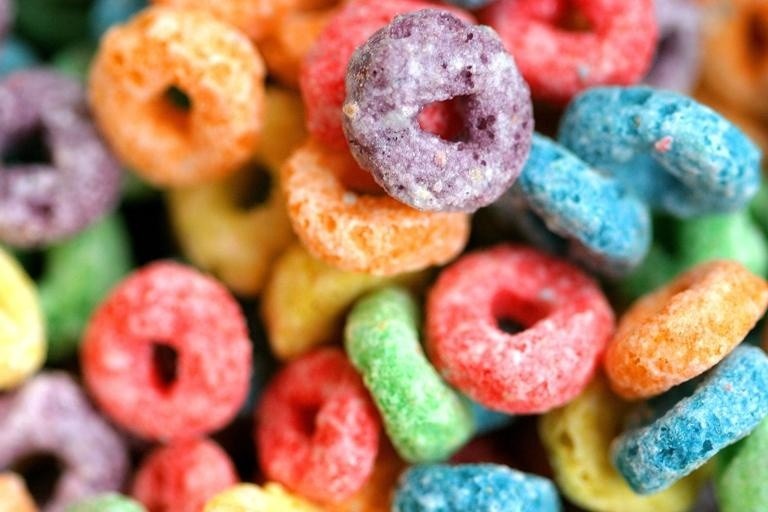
left=0, top=0, right=768, bottom=512
